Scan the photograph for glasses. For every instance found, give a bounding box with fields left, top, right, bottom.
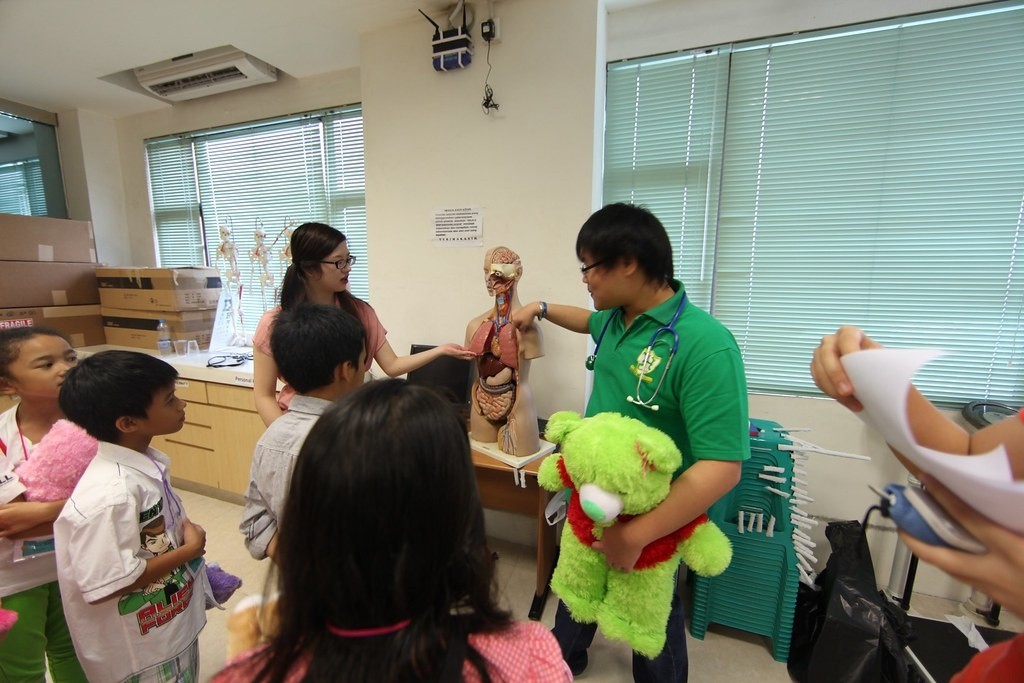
left=581, top=260, right=609, bottom=274
left=318, top=254, right=357, bottom=269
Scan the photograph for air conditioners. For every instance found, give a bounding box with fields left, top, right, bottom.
left=132, top=44, right=277, bottom=102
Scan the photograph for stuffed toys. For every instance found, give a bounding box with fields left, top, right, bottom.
left=538, top=411, right=730, bottom=661
left=203, top=561, right=241, bottom=609
left=15, top=417, right=98, bottom=505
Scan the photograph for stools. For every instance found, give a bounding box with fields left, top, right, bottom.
left=690, top=419, right=801, bottom=663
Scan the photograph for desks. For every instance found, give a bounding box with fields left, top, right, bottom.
left=467, top=421, right=561, bottom=620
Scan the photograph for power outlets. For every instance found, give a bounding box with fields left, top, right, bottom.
left=480, top=16, right=502, bottom=47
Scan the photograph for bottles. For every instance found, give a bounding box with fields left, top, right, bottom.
left=157, top=319, right=171, bottom=356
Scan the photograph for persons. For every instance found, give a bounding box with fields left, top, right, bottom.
left=215, top=225, right=294, bottom=345
left=53, top=351, right=224, bottom=682
left=252, top=224, right=476, bottom=428
left=240, top=305, right=368, bottom=560
left=210, top=378, right=573, bottom=683
left=463, top=247, right=545, bottom=456
left=0, top=327, right=90, bottom=682
left=811, top=325, right=1024, bottom=683
left=511, top=203, right=753, bottom=682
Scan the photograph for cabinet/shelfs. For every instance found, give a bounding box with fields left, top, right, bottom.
left=149, top=376, right=286, bottom=497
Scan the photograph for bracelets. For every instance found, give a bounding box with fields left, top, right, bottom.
left=538, top=300, right=547, bottom=320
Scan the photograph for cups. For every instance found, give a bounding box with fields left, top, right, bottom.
left=188, top=340, right=200, bottom=358
left=174, top=339, right=186, bottom=357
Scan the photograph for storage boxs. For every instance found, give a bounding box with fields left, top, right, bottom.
left=0, top=213, right=222, bottom=351
